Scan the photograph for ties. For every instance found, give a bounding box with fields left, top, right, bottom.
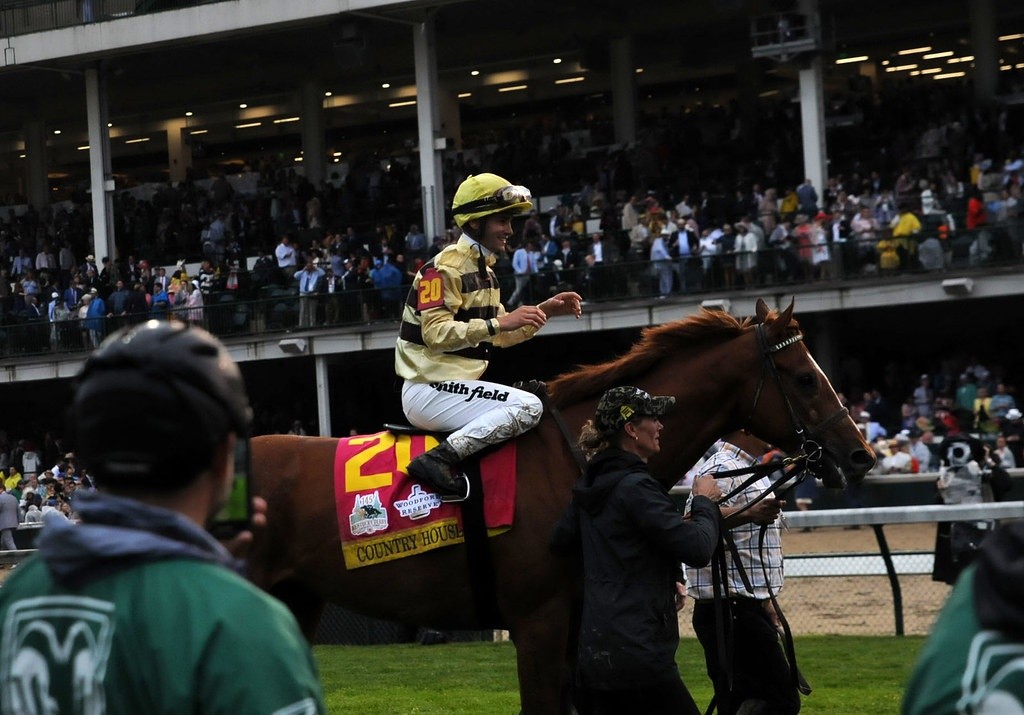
left=161, top=276, right=164, bottom=285
left=527, top=255, right=532, bottom=273
left=304, top=272, right=311, bottom=292
left=72, top=288, right=75, bottom=301
left=45, top=254, right=51, bottom=268
left=328, top=277, right=332, bottom=285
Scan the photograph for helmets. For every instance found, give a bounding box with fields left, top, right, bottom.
left=73, top=319, right=248, bottom=440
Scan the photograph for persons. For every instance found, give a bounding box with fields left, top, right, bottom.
left=567, top=386, right=721, bottom=715
left=393, top=172, right=582, bottom=497
left=899, top=515, right=1024, bottom=715
left=684, top=426, right=801, bottom=715
left=0, top=81, right=1024, bottom=566
left=0, top=320, right=325, bottom=715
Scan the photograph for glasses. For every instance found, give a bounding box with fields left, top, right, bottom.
left=491, top=185, right=533, bottom=206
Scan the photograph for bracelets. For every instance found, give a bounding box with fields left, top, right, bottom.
left=486, top=320, right=495, bottom=336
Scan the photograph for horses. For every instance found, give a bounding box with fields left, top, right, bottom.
left=202, top=296, right=878, bottom=715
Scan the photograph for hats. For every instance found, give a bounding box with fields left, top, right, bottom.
left=915, top=416, right=935, bottom=432
left=90, top=287, right=97, bottom=294
left=898, top=202, right=909, bottom=210
left=64, top=453, right=75, bottom=459
left=594, top=386, right=676, bottom=436
left=1004, top=408, right=1021, bottom=420
left=85, top=254, right=95, bottom=261
left=734, top=222, right=749, bottom=231
left=452, top=172, right=533, bottom=229
left=191, top=279, right=200, bottom=289
left=44, top=470, right=54, bottom=476
left=51, top=292, right=59, bottom=299
left=816, top=212, right=833, bottom=220
left=793, top=214, right=809, bottom=224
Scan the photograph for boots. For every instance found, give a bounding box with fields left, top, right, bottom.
left=406, top=440, right=460, bottom=493
